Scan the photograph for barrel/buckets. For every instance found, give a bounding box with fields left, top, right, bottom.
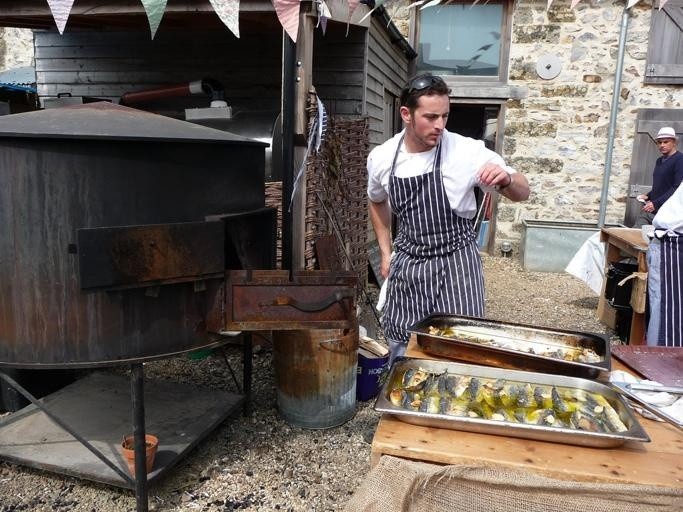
left=273, top=327, right=357, bottom=428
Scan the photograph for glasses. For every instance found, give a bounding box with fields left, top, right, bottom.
left=405, top=76, right=442, bottom=90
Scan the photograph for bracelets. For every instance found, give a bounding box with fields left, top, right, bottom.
left=494, top=172, right=511, bottom=191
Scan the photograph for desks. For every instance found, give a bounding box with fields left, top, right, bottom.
left=368, top=332, right=683, bottom=490
left=593, top=228, right=656, bottom=346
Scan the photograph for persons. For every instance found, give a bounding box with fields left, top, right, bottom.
left=365, top=72, right=530, bottom=353
left=632, top=125, right=683, bottom=227
left=645, top=181, right=683, bottom=347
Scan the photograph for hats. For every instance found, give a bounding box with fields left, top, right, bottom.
left=655, top=127, right=679, bottom=142
